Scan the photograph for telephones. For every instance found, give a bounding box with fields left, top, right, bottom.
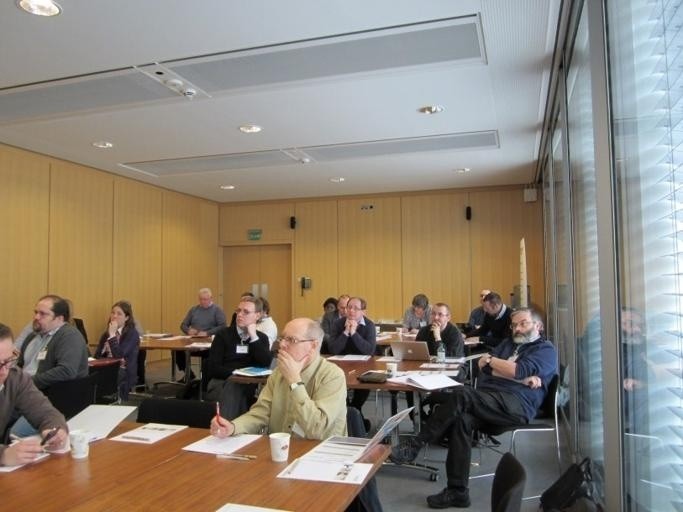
left=302, top=276, right=311, bottom=289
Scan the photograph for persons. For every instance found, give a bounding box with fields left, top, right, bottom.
left=174, top=288, right=225, bottom=384
left=10, top=295, right=88, bottom=439
left=210, top=318, right=348, bottom=441
left=93, top=301, right=140, bottom=395
left=621, top=306, right=659, bottom=426
left=388, top=308, right=558, bottom=510
left=401, top=287, right=514, bottom=379
left=0, top=323, right=70, bottom=465
left=206, top=292, right=278, bottom=419
left=319, top=294, right=376, bottom=412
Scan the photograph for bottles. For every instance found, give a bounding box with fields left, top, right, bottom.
left=436, top=342, right=445, bottom=362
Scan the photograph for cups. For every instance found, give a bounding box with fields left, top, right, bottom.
left=69, top=428, right=89, bottom=459
left=395, top=327, right=402, bottom=335
left=268, top=433, right=290, bottom=461
left=385, top=363, right=397, bottom=378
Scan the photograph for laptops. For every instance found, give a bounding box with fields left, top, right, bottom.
left=391, top=341, right=430, bottom=361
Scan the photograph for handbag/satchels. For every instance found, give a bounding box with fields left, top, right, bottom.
left=540, top=457, right=605, bottom=511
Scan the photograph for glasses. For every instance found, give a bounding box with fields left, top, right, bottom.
left=277, top=335, right=315, bottom=346
left=510, top=321, right=538, bottom=329
left=0, top=351, right=19, bottom=369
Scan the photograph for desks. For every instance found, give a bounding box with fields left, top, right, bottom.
left=89, top=329, right=213, bottom=384
left=228, top=355, right=471, bottom=484
left=373, top=323, right=478, bottom=419
left=0, top=414, right=391, bottom=511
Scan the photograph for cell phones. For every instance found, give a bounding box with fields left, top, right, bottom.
left=40, top=426, right=60, bottom=445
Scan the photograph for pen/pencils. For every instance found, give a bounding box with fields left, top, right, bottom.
left=348, top=369, right=356, bottom=374
left=216, top=401, right=221, bottom=425
left=9, top=433, right=49, bottom=454
left=216, top=452, right=257, bottom=461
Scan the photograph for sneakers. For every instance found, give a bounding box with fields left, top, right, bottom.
left=388, top=438, right=422, bottom=464
left=178, top=372, right=196, bottom=383
left=427, top=488, right=471, bottom=509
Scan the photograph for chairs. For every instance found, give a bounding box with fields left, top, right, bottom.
left=40, top=356, right=125, bottom=421
left=134, top=398, right=221, bottom=430
left=469, top=372, right=561, bottom=502
